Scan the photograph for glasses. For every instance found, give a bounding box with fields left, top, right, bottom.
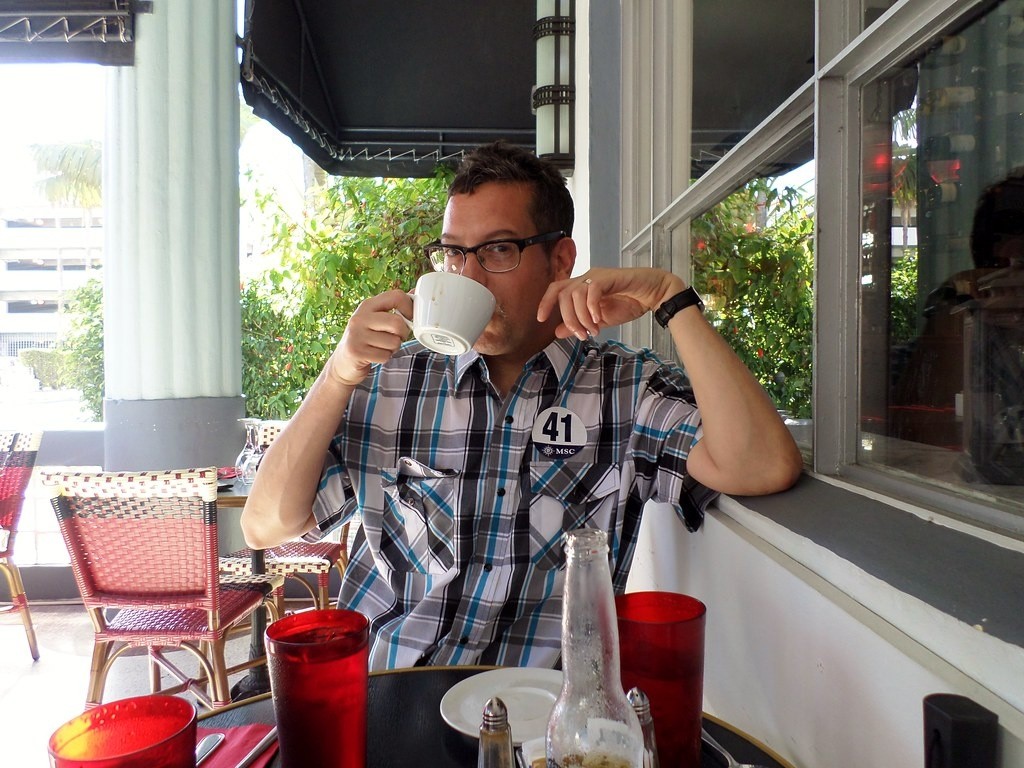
left=423, top=231, right=568, bottom=277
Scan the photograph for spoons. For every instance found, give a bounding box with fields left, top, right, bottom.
left=701, top=727, right=758, bottom=768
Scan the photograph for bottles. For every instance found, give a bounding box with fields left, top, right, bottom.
left=235, top=422, right=264, bottom=485
left=627, top=687, right=659, bottom=768
left=478, top=697, right=516, bottom=768
left=544, top=528, right=643, bottom=768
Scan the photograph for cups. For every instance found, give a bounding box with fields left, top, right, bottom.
left=264, top=609, right=370, bottom=768
left=394, top=272, right=496, bottom=355
left=611, top=591, right=705, bottom=768
left=49, top=695, right=197, bottom=768
left=439, top=666, right=563, bottom=743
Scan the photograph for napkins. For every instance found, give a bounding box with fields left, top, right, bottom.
left=196, top=723, right=279, bottom=768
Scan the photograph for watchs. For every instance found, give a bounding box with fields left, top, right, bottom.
left=655, top=285, right=704, bottom=330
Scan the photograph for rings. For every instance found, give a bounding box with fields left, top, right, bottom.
left=582, top=279, right=592, bottom=284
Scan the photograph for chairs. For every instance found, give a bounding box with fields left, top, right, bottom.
left=0, top=429, right=48, bottom=662
left=41, top=465, right=285, bottom=715
left=219, top=419, right=349, bottom=615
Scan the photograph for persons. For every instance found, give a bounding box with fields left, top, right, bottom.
left=864, top=173, right=1024, bottom=469
left=240, top=140, right=803, bottom=669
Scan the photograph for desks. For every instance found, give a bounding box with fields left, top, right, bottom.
left=194, top=666, right=795, bottom=768
left=218, top=473, right=272, bottom=703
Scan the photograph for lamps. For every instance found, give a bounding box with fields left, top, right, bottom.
left=532, top=0, right=576, bottom=177
